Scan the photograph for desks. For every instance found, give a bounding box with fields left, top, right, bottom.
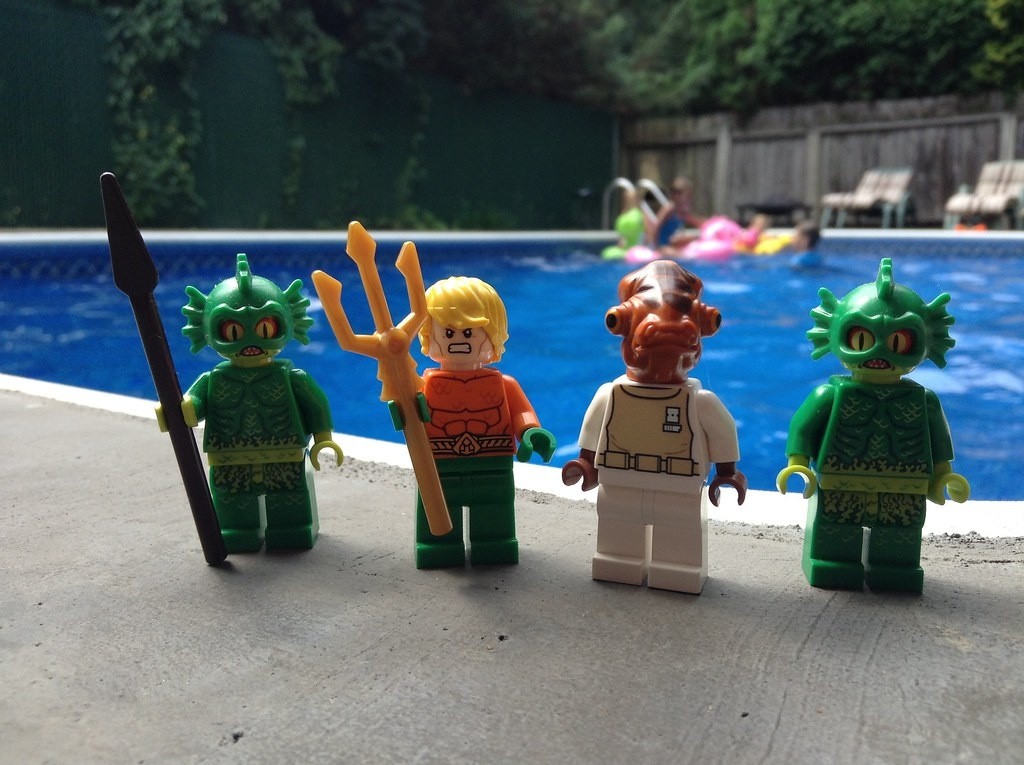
left=736, top=200, right=811, bottom=228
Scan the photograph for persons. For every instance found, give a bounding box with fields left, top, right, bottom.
left=615, top=173, right=831, bottom=275
left=153, top=253, right=344, bottom=554
left=558, top=260, right=749, bottom=596
left=773, top=258, right=972, bottom=596
left=381, top=268, right=558, bottom=574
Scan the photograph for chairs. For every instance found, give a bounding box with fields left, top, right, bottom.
left=820, top=167, right=915, bottom=229
left=944, top=159, right=1023, bottom=230
